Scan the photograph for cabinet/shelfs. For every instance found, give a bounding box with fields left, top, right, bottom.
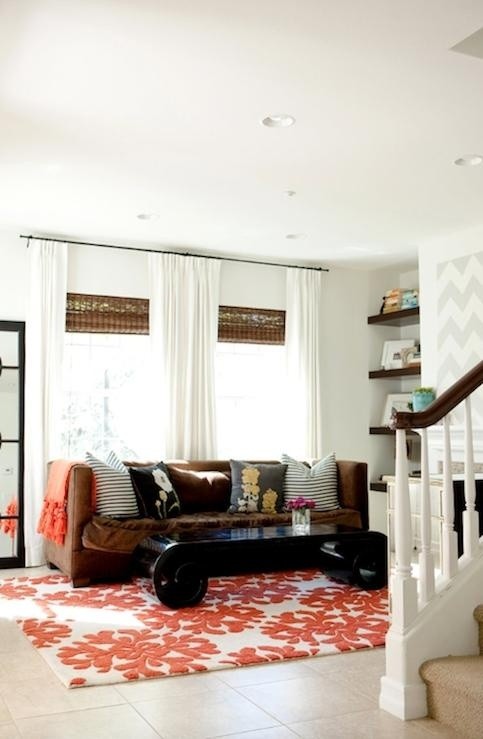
left=368, top=307, right=420, bottom=492
left=380, top=474, right=483, bottom=618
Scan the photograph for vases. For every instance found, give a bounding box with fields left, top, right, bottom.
left=292, top=508, right=311, bottom=525
left=42, top=458, right=369, bottom=588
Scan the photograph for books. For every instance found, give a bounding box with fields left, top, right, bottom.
left=382, top=338, right=421, bottom=371
left=383, top=287, right=418, bottom=316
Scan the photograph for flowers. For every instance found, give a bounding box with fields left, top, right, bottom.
left=237, top=466, right=278, bottom=514
left=152, top=469, right=180, bottom=519
left=286, top=496, right=316, bottom=515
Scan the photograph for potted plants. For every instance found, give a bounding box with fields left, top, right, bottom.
left=412, top=388, right=436, bottom=414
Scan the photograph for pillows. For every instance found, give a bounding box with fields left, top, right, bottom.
left=281, top=453, right=342, bottom=513
left=166, top=465, right=232, bottom=513
left=85, top=450, right=140, bottom=521
left=226, top=459, right=288, bottom=514
left=125, top=461, right=183, bottom=521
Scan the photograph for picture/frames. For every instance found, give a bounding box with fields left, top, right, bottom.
left=381, top=339, right=415, bottom=371
left=382, top=392, right=416, bottom=428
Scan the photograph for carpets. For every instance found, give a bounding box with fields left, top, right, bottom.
left=0, top=568, right=393, bottom=690
